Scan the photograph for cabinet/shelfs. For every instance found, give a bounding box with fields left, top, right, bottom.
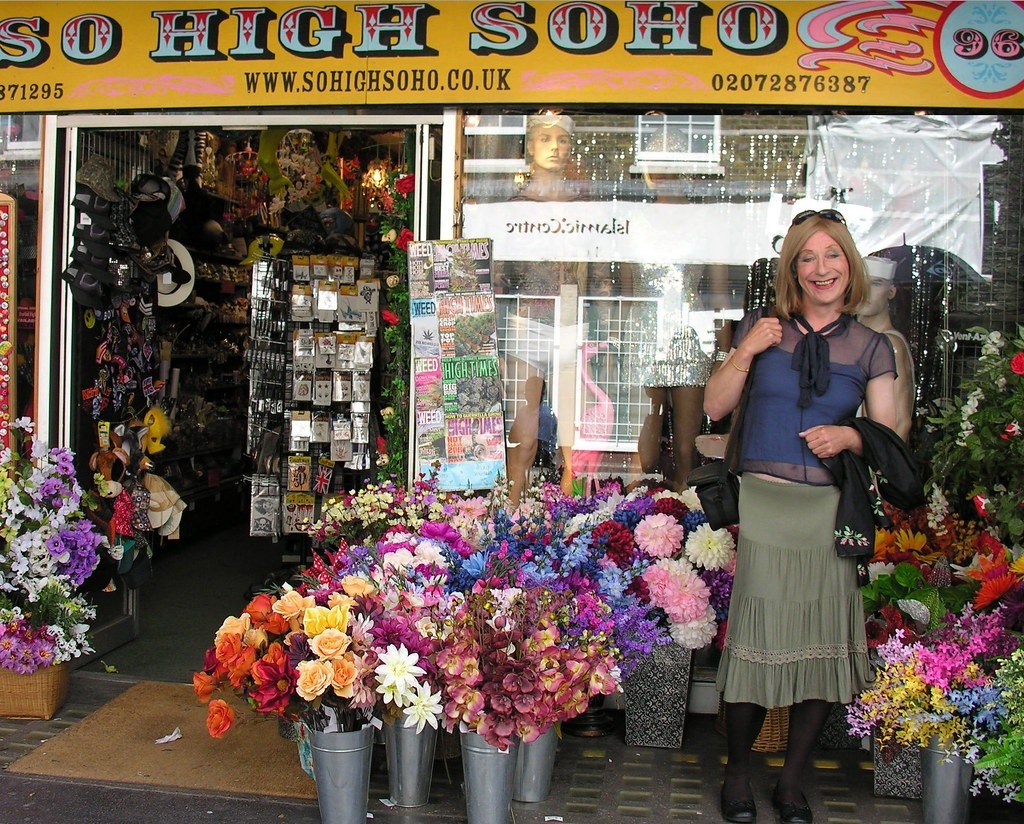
left=153, top=185, right=251, bottom=503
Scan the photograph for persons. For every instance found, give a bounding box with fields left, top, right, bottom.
left=704, top=209, right=897, bottom=824
left=491, top=108, right=918, bottom=505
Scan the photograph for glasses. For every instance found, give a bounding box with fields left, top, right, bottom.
left=788, top=209, right=846, bottom=227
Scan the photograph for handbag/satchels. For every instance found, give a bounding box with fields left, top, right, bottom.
left=686, top=461, right=741, bottom=531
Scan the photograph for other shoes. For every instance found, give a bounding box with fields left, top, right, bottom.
left=772, top=779, right=813, bottom=824
left=720, top=785, right=758, bottom=822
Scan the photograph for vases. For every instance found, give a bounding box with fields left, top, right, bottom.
left=813, top=702, right=862, bottom=751
left=624, top=639, right=695, bottom=748
left=875, top=726, right=974, bottom=824
left=279, top=713, right=558, bottom=824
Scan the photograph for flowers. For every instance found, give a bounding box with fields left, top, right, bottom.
left=843, top=321, right=1024, bottom=804
left=0, top=416, right=112, bottom=674
left=374, top=166, right=415, bottom=481
left=192, top=458, right=738, bottom=750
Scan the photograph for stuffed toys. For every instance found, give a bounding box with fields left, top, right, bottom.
left=90, top=405, right=186, bottom=576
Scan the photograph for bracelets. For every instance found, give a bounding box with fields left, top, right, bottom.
left=729, top=357, right=750, bottom=372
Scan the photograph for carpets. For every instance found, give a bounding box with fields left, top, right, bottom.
left=5, top=681, right=317, bottom=800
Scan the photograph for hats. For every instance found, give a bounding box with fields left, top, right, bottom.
left=85, top=471, right=187, bottom=595
left=239, top=207, right=363, bottom=264
left=59, top=154, right=197, bottom=317
